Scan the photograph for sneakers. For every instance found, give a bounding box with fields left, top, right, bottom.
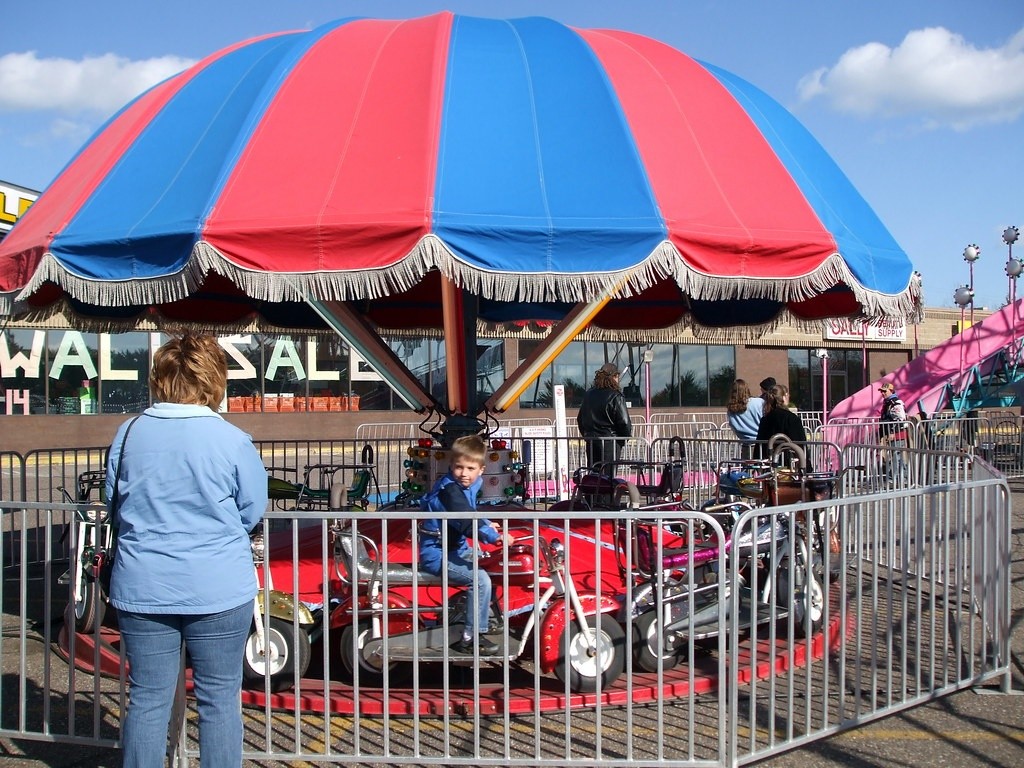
left=487, top=619, right=503, bottom=635
left=457, top=630, right=500, bottom=655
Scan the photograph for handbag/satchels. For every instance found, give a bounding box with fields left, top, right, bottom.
left=99, top=556, right=114, bottom=594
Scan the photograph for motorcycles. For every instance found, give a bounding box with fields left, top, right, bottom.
left=326, top=527, right=628, bottom=690
left=697, top=452, right=870, bottom=585
left=55, top=485, right=313, bottom=695
left=611, top=494, right=825, bottom=672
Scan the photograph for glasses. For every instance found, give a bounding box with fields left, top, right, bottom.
left=881, top=390, right=887, bottom=393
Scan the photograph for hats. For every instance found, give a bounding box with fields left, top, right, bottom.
left=760, top=378, right=776, bottom=390
left=601, top=364, right=618, bottom=375
left=878, top=383, right=894, bottom=391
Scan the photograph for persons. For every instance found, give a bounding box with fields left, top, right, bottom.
left=728, top=377, right=813, bottom=473
left=875, top=382, right=907, bottom=462
left=577, top=363, right=632, bottom=477
left=420, top=435, right=514, bottom=655
left=104, top=329, right=268, bottom=768
